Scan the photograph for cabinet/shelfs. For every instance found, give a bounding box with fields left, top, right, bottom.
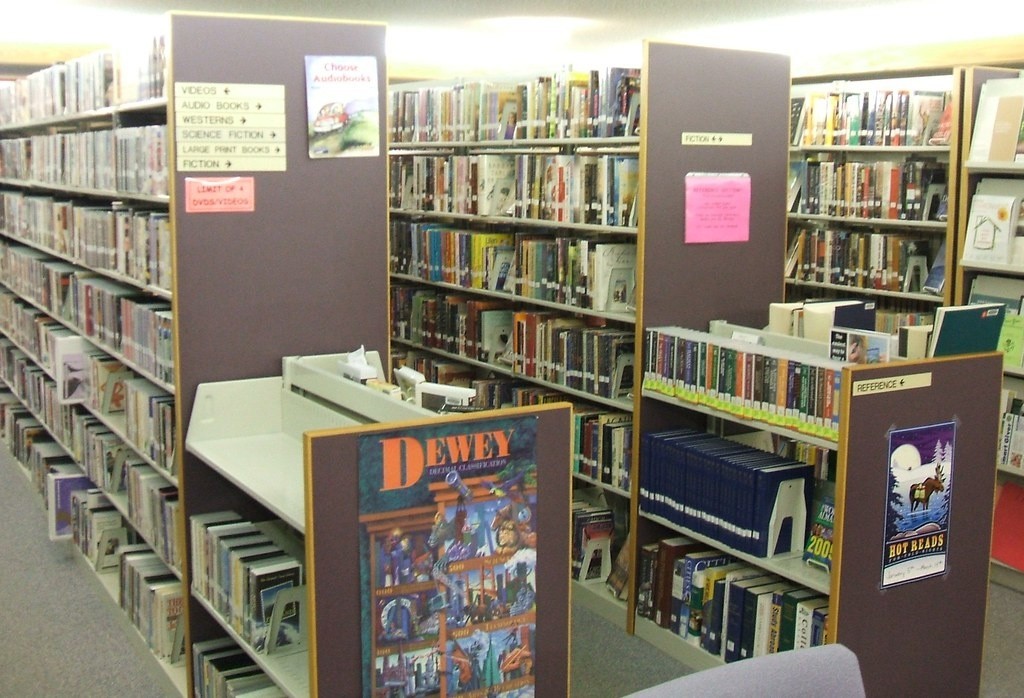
left=635, top=293, right=1008, bottom=698
left=184, top=343, right=574, bottom=698
left=390, top=36, right=793, bottom=634
left=953, top=65, right=1024, bottom=594
left=785, top=67, right=963, bottom=335
left=0, top=9, right=392, bottom=698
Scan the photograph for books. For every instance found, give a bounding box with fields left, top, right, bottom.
left=0, top=31, right=1024, bottom=698
